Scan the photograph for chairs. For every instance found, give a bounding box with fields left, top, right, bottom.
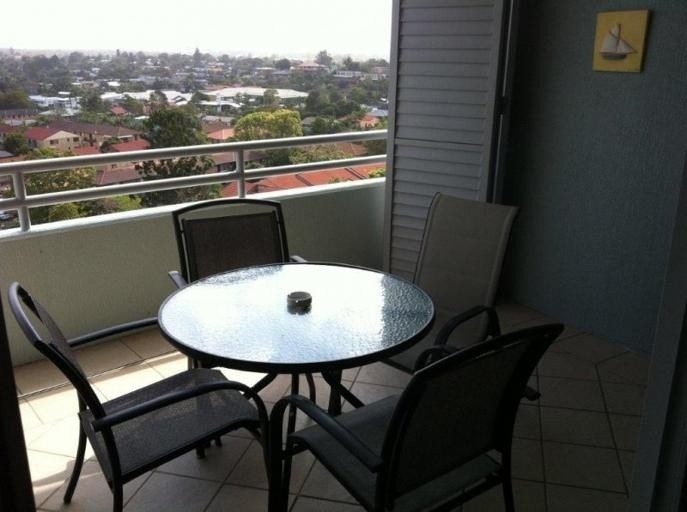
left=163, top=196, right=308, bottom=457
left=321, top=192, right=541, bottom=411
left=8, top=278, right=270, bottom=512
left=269, top=317, right=563, bottom=509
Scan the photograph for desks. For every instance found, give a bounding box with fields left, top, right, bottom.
left=156, top=264, right=434, bottom=511
